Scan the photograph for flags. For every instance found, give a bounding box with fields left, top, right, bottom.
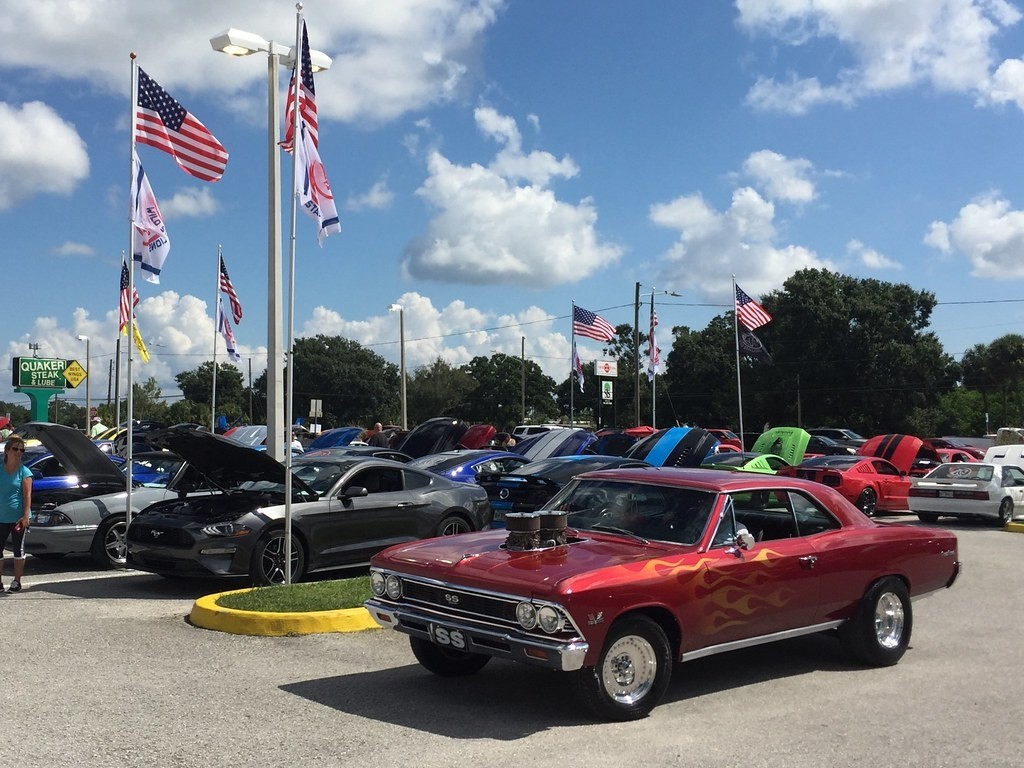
left=121, top=317, right=150, bottom=365
left=135, top=67, right=227, bottom=183
left=220, top=253, right=242, bottom=325
left=297, top=113, right=341, bottom=249
left=276, top=19, right=318, bottom=155
left=572, top=305, right=615, bottom=342
left=119, top=261, right=139, bottom=330
left=735, top=283, right=772, bottom=331
left=649, top=295, right=660, bottom=383
left=218, top=298, right=242, bottom=364
left=571, top=340, right=585, bottom=394
left=131, top=151, right=170, bottom=285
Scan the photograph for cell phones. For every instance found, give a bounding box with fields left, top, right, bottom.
left=15, top=522, right=23, bottom=533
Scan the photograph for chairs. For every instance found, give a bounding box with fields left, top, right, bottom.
left=353, top=471, right=379, bottom=493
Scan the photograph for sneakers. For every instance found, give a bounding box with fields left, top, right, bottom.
left=0, top=583, right=5, bottom=593
left=7, top=580, right=22, bottom=594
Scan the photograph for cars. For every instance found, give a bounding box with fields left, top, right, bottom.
left=362, top=466, right=962, bottom=723
left=125, top=426, right=495, bottom=588
left=907, top=444, right=1024, bottom=528
left=772, top=434, right=925, bottom=520
left=0, top=414, right=868, bottom=570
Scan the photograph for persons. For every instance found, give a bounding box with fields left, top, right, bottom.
left=681, top=494, right=755, bottom=546
left=283, top=429, right=304, bottom=458
left=90, top=417, right=108, bottom=438
left=368, top=422, right=389, bottom=448
left=0, top=436, right=33, bottom=594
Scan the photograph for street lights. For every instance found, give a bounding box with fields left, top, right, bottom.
left=209, top=28, right=334, bottom=462
left=386, top=304, right=408, bottom=431
left=76, top=334, right=90, bottom=435
left=635, top=282, right=685, bottom=439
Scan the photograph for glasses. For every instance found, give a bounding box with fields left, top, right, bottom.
left=10, top=446, right=25, bottom=453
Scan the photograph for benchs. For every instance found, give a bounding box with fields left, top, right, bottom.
left=734, top=511, right=828, bottom=537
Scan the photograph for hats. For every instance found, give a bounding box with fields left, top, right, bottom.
left=91, top=417, right=103, bottom=421
